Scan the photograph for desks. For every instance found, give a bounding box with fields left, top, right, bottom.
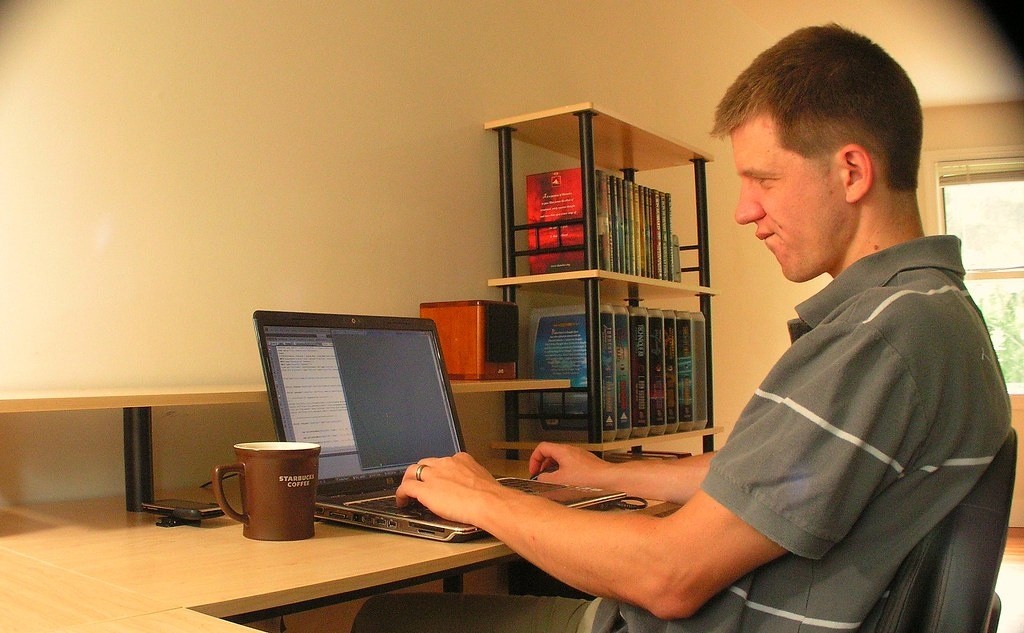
left=1, top=380, right=671, bottom=633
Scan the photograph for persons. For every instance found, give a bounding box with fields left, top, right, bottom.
left=396, top=21, right=1012, bottom=633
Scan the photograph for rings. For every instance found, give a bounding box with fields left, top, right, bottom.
left=415, top=464, right=429, bottom=482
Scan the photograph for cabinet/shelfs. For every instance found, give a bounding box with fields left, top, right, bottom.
left=485, top=102, right=723, bottom=459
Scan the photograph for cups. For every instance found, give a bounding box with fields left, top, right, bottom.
left=211, top=442, right=320, bottom=540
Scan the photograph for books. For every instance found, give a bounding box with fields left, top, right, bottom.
left=525, top=168, right=682, bottom=282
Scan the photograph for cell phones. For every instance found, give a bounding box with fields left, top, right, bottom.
left=141, top=499, right=224, bottom=520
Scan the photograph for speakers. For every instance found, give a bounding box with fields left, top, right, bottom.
left=420, top=300, right=518, bottom=381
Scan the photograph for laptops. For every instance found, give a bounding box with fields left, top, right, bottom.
left=253, top=310, right=626, bottom=543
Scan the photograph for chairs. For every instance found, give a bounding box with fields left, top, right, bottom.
left=870, top=426, right=1017, bottom=633
